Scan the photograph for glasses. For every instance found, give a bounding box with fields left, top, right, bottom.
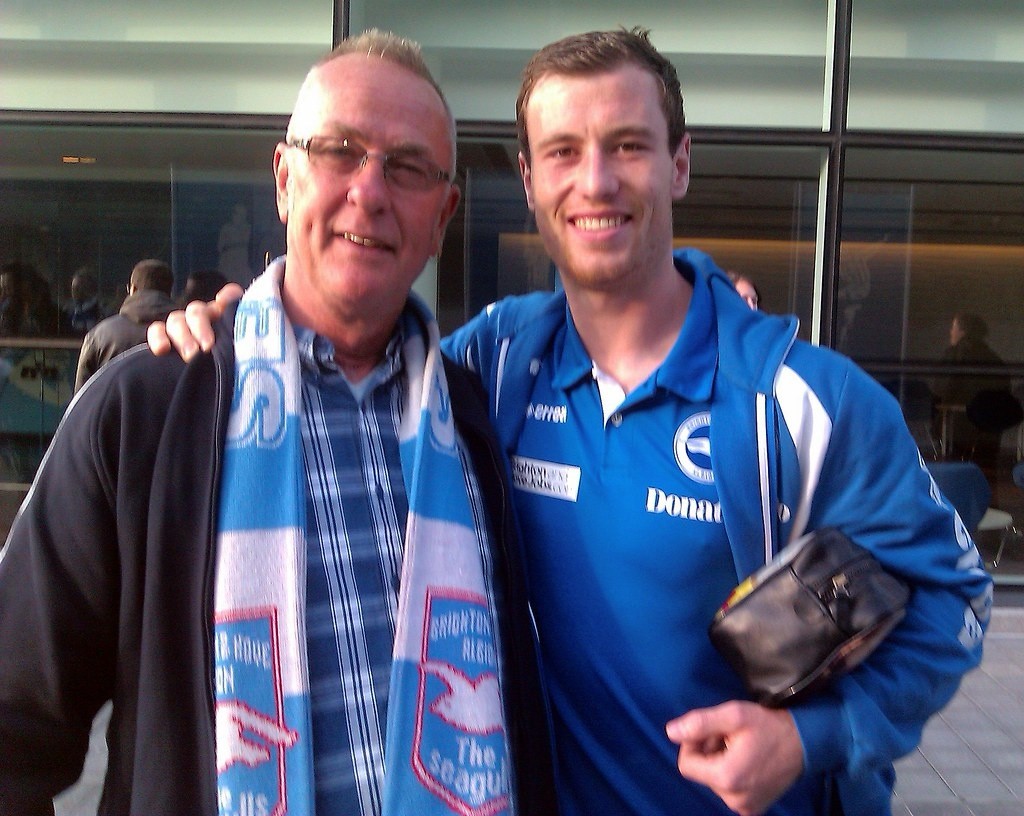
left=126, top=284, right=130, bottom=293
left=287, top=135, right=450, bottom=191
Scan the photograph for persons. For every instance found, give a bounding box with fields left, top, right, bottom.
left=0, top=261, right=71, bottom=481
left=76, top=260, right=229, bottom=396
left=728, top=272, right=758, bottom=313
left=59, top=271, right=108, bottom=377
left=147, top=29, right=995, bottom=816
left=0, top=31, right=567, bottom=816
left=837, top=302, right=934, bottom=460
left=934, top=311, right=1011, bottom=480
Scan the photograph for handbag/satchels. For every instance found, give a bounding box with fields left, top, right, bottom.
left=713, top=527, right=909, bottom=705
